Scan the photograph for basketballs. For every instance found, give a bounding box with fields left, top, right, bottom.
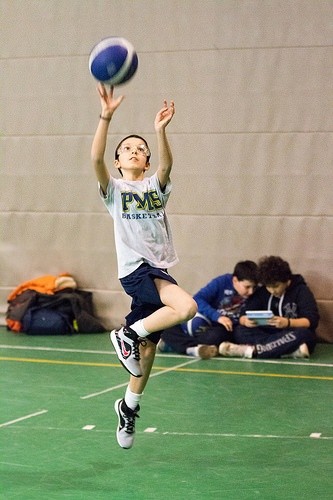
left=89, top=37, right=138, bottom=85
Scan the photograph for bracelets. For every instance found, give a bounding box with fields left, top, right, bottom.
left=99, top=115, right=112, bottom=120
left=287, top=318, right=291, bottom=328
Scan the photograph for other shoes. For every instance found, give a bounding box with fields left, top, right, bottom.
left=219, top=342, right=255, bottom=361
left=294, top=342, right=310, bottom=358
left=196, top=344, right=218, bottom=359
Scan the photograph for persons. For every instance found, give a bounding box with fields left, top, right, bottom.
left=157, top=255, right=320, bottom=361
left=92, top=82, right=198, bottom=449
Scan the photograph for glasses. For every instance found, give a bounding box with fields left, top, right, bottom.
left=116, top=145, right=152, bottom=156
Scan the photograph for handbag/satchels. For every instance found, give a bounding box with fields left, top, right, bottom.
left=20, top=297, right=79, bottom=335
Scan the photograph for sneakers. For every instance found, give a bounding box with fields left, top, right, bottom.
left=110, top=326, right=143, bottom=377
left=114, top=398, right=140, bottom=449
